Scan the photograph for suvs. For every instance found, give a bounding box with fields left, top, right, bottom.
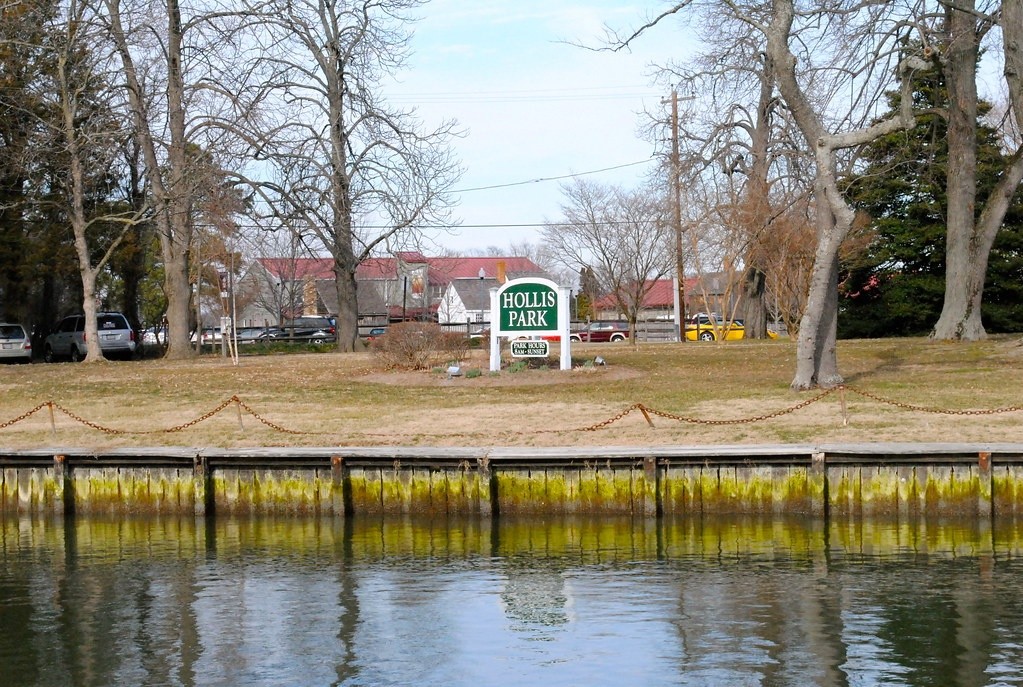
left=0, top=324, right=31, bottom=362
left=570, top=319, right=638, bottom=343
left=44, top=311, right=136, bottom=364
left=256, top=315, right=337, bottom=346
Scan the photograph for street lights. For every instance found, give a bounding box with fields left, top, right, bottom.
left=276, top=275, right=281, bottom=325
left=479, top=268, right=485, bottom=328
left=402, top=269, right=408, bottom=322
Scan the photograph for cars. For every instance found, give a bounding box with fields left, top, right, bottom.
left=367, top=327, right=386, bottom=342
left=692, top=313, right=717, bottom=323
left=141, top=324, right=223, bottom=349
left=470, top=326, right=490, bottom=345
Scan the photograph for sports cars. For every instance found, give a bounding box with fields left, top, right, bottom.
left=686, top=317, right=778, bottom=342
